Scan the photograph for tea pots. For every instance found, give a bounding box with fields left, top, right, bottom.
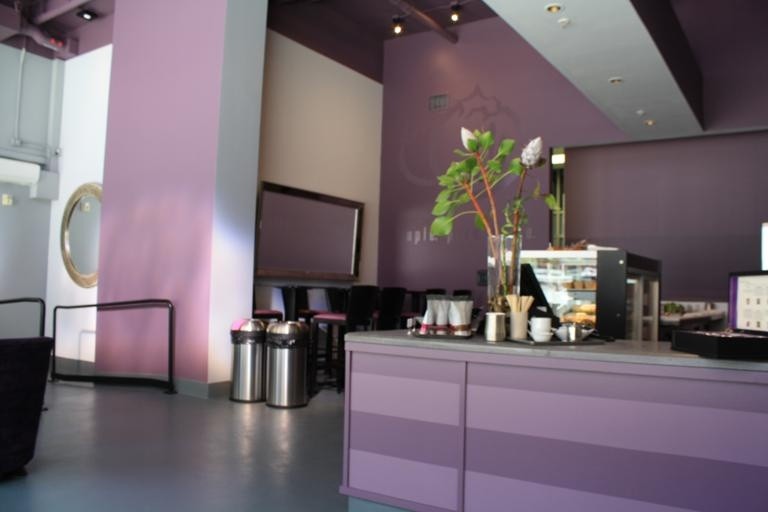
left=552, top=321, right=596, bottom=344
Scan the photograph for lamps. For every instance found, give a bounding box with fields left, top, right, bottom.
left=392, top=15, right=407, bottom=35
left=449, top=2, right=462, bottom=22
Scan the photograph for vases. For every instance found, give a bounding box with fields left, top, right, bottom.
left=482, top=233, right=522, bottom=312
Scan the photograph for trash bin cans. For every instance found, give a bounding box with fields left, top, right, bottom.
left=229, top=319, right=311, bottom=409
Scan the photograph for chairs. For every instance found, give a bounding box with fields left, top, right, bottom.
left=252, top=282, right=471, bottom=395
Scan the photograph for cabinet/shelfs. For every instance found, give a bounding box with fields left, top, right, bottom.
left=519, top=247, right=617, bottom=324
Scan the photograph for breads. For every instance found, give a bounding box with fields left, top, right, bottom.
left=563, top=281, right=597, bottom=290
left=564, top=303, right=596, bottom=326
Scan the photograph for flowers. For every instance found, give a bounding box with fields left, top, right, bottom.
left=428, top=123, right=561, bottom=297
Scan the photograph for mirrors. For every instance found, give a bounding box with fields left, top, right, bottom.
left=255, top=179, right=366, bottom=284
left=58, top=182, right=104, bottom=291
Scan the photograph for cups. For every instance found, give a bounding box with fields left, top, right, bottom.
left=528, top=316, right=553, bottom=343
left=426, top=294, right=473, bottom=336
left=512, top=312, right=527, bottom=339
left=485, top=311, right=507, bottom=342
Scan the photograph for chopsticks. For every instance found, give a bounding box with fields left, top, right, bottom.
left=506, top=293, right=535, bottom=312
